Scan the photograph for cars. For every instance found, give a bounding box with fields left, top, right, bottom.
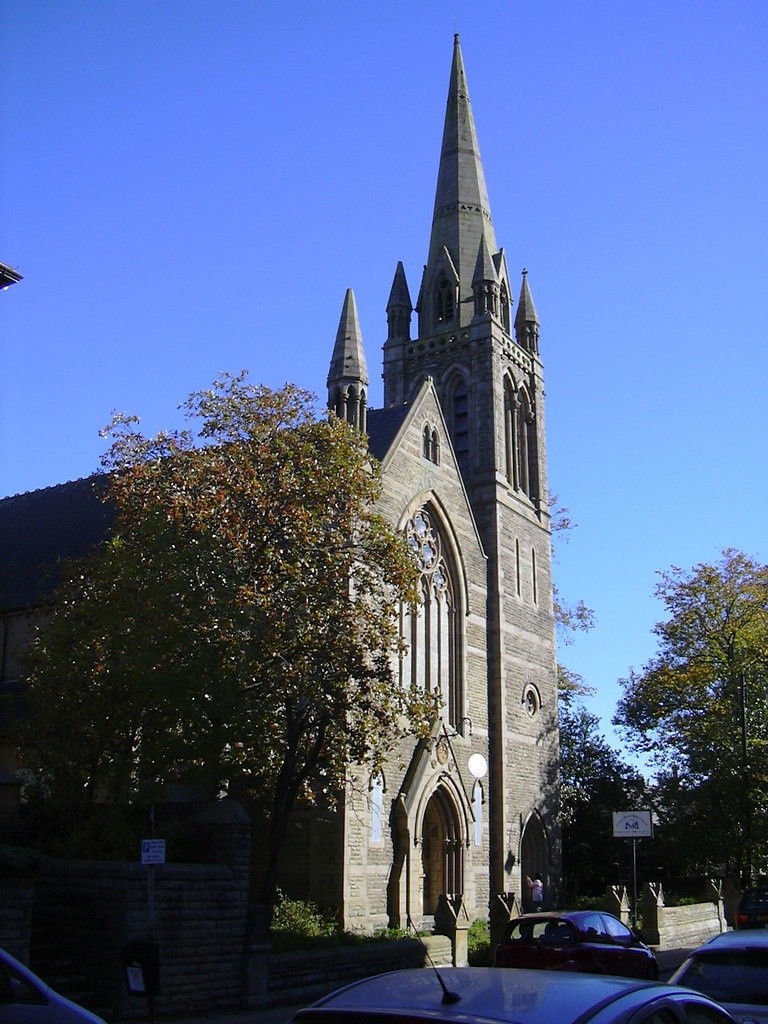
left=285, top=965, right=739, bottom=1024
left=735, top=886, right=768, bottom=928
left=667, top=927, right=768, bottom=1024
left=0, top=944, right=109, bottom=1024
left=491, top=909, right=661, bottom=979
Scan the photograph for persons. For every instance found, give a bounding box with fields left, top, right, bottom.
left=525, top=872, right=544, bottom=913
